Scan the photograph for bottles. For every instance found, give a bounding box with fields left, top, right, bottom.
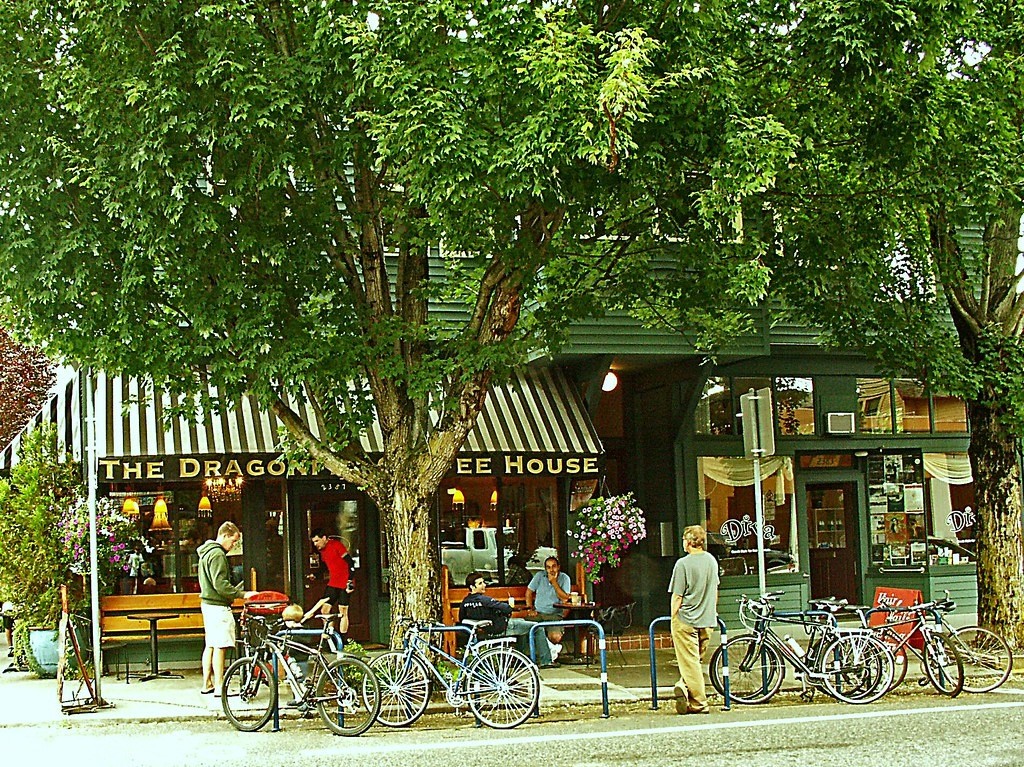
left=784, top=634, right=806, bottom=657
left=286, top=655, right=303, bottom=679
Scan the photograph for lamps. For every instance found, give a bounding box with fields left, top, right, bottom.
left=855, top=451, right=869, bottom=456
left=122, top=484, right=141, bottom=520
left=490, top=490, right=498, bottom=510
left=148, top=481, right=172, bottom=532
left=197, top=485, right=213, bottom=518
left=601, top=370, right=618, bottom=391
left=334, top=482, right=340, bottom=490
left=321, top=485, right=327, bottom=491
left=328, top=484, right=332, bottom=490
left=447, top=478, right=456, bottom=494
left=341, top=482, right=345, bottom=490
left=452, top=489, right=464, bottom=510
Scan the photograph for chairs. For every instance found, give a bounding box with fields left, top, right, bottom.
left=74, top=615, right=127, bottom=681
left=454, top=606, right=524, bottom=667
left=586, top=601, right=639, bottom=669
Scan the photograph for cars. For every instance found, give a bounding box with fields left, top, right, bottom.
left=729, top=549, right=796, bottom=575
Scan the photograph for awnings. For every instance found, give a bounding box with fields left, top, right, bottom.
left=95, top=364, right=607, bottom=483
left=0, top=367, right=82, bottom=475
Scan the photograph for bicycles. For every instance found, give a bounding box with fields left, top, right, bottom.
left=221, top=602, right=382, bottom=737
left=362, top=615, right=545, bottom=730
left=709, top=590, right=1013, bottom=705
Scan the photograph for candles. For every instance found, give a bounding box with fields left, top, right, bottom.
left=506, top=519, right=510, bottom=527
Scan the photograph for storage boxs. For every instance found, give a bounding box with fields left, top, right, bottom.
left=928, top=547, right=968, bottom=566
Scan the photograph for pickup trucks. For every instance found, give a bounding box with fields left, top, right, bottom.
left=441, top=526, right=557, bottom=588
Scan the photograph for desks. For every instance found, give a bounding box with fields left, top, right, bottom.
left=127, top=613, right=185, bottom=683
left=553, top=602, right=600, bottom=665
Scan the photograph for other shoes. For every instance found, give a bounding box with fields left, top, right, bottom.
left=200, top=687, right=214, bottom=693
left=541, top=662, right=560, bottom=669
left=690, top=709, right=709, bottom=714
left=675, top=686, right=687, bottom=714
left=214, top=688, right=239, bottom=697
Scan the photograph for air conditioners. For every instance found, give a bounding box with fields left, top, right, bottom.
left=824, top=413, right=856, bottom=434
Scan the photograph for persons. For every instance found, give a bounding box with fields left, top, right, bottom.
left=667, top=525, right=720, bottom=715
left=197, top=521, right=260, bottom=697
left=310, top=528, right=356, bottom=649
left=507, top=556, right=533, bottom=584
left=458, top=573, right=561, bottom=669
left=525, top=557, right=571, bottom=645
left=2, top=601, right=15, bottom=657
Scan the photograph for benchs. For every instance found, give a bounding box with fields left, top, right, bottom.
left=100, top=567, right=257, bottom=675
left=443, top=562, right=588, bottom=663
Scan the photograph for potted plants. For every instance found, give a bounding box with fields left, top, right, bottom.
left=0, top=418, right=97, bottom=681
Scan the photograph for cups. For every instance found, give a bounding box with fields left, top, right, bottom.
left=571, top=592, right=579, bottom=603
left=509, top=597, right=514, bottom=608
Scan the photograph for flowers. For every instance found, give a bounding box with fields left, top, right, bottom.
left=48, top=495, right=154, bottom=575
left=566, top=490, right=647, bottom=584
